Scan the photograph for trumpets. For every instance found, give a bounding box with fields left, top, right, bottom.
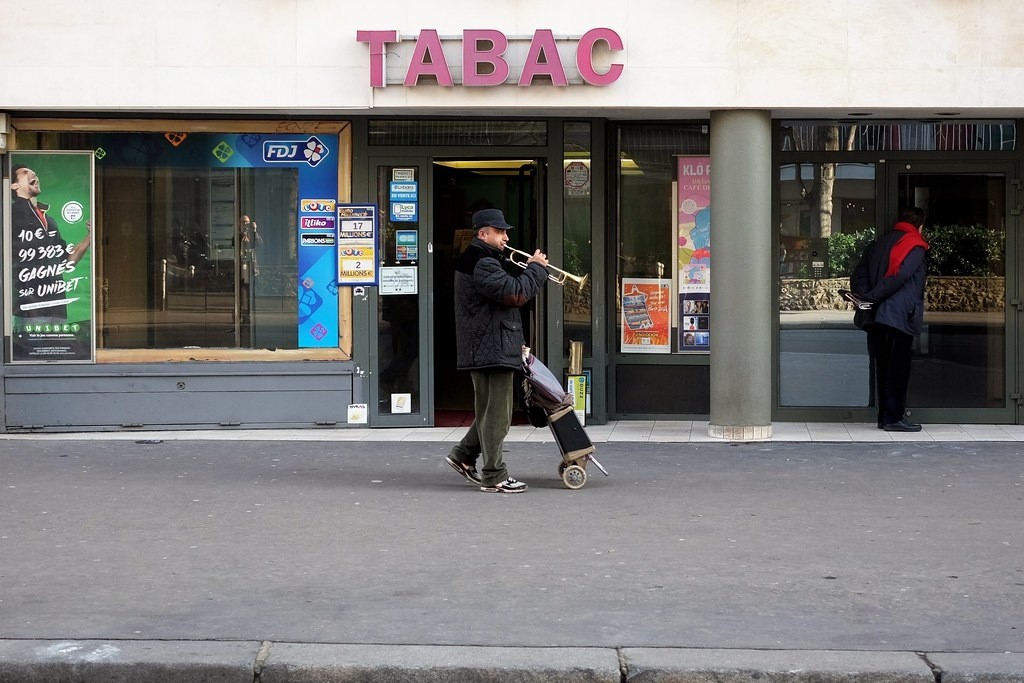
left=502, top=244, right=589, bottom=294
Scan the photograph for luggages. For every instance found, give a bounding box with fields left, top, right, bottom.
left=521, top=352, right=608, bottom=489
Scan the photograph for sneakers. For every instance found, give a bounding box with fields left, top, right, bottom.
left=480, top=475, right=529, bottom=493
left=444, top=454, right=482, bottom=486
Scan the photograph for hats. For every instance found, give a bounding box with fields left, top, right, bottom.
left=472, top=209, right=514, bottom=230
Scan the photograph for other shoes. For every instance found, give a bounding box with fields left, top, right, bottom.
left=877, top=417, right=915, bottom=429
left=884, top=417, right=922, bottom=432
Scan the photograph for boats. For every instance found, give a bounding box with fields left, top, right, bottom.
left=631, top=320, right=649, bottom=325
left=628, top=309, right=647, bottom=314
left=625, top=303, right=645, bottom=307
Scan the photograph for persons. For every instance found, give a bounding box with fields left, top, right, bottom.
left=684, top=301, right=708, bottom=345
left=444, top=208, right=550, bottom=494
left=850, top=206, right=929, bottom=432
left=225, top=214, right=263, bottom=335
left=10, top=164, right=92, bottom=329
